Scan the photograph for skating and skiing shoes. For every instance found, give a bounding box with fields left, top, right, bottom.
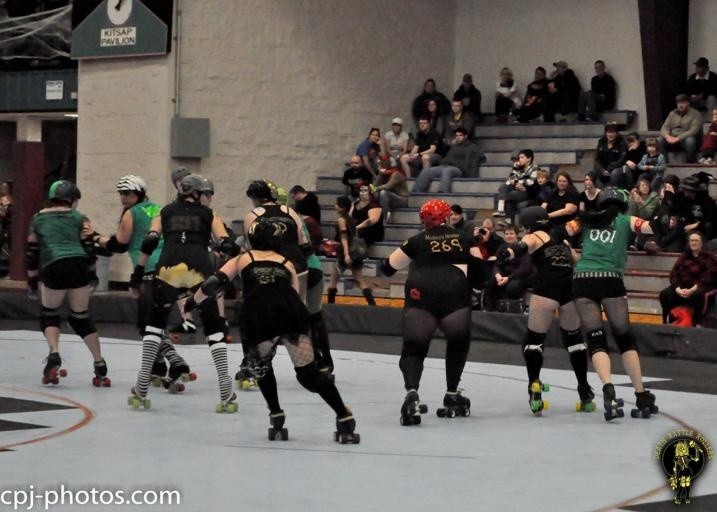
left=632, top=390, right=658, bottom=418
left=268, top=411, right=288, bottom=440
left=92, top=361, right=111, bottom=387
left=216, top=393, right=237, bottom=413
left=437, top=390, right=471, bottom=417
left=575, top=384, right=596, bottom=411
left=334, top=414, right=359, bottom=444
left=41, top=352, right=67, bottom=384
left=400, top=389, right=427, bottom=424
left=528, top=379, right=549, bottom=416
left=602, top=384, right=624, bottom=420
left=127, top=362, right=196, bottom=411
left=235, top=370, right=258, bottom=390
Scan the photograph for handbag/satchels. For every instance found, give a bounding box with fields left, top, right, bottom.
left=349, top=236, right=368, bottom=260
left=671, top=306, right=694, bottom=327
left=496, top=298, right=526, bottom=313
left=320, top=238, right=339, bottom=256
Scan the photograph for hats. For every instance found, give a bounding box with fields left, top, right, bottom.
left=553, top=62, right=567, bottom=67
left=694, top=58, right=708, bottom=66
left=392, top=117, right=402, bottom=124
left=679, top=176, right=704, bottom=191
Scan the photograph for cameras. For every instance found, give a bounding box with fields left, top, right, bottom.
left=479, top=229, right=485, bottom=234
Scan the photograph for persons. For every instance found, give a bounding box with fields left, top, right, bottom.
left=0, top=179, right=14, bottom=279
left=495, top=206, right=596, bottom=412
left=128, top=172, right=235, bottom=403
left=179, top=221, right=356, bottom=433
left=176, top=59, right=717, bottom=326
left=26, top=181, right=107, bottom=380
left=378, top=200, right=486, bottom=414
left=234, top=180, right=312, bottom=380
left=277, top=188, right=334, bottom=373
left=554, top=186, right=688, bottom=412
left=83, top=175, right=189, bottom=382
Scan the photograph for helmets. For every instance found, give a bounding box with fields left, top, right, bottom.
left=49, top=181, right=81, bottom=206
left=248, top=216, right=287, bottom=248
left=418, top=199, right=450, bottom=230
left=116, top=174, right=146, bottom=196
left=597, top=186, right=629, bottom=210
left=518, top=206, right=547, bottom=226
left=171, top=167, right=214, bottom=196
left=246, top=179, right=288, bottom=205
